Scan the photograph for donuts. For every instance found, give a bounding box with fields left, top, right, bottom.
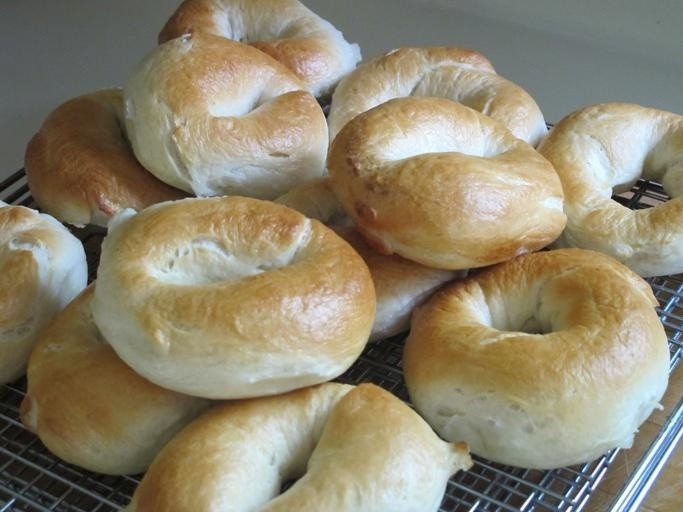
left=1, top=0, right=682, bottom=512
